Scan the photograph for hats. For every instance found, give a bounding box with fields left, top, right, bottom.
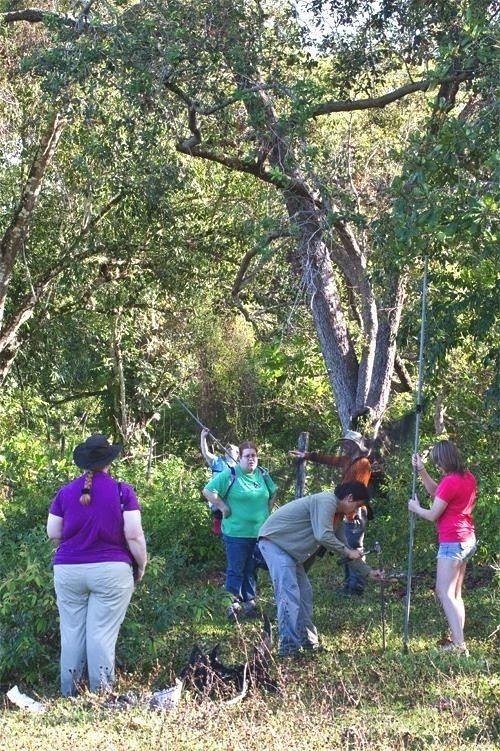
left=73, top=434, right=122, bottom=469
left=335, top=430, right=368, bottom=452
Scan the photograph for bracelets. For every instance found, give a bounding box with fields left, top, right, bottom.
left=417, top=465, right=425, bottom=471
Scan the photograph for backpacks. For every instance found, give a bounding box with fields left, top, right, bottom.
left=212, top=466, right=266, bottom=536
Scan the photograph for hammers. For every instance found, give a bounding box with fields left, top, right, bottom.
left=337, top=541, right=382, bottom=565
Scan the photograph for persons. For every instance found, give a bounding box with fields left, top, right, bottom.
left=257, top=479, right=385, bottom=656
left=408, top=440, right=477, bottom=655
left=288, top=430, right=371, bottom=595
left=47, top=434, right=147, bottom=696
left=200, top=427, right=278, bottom=612
